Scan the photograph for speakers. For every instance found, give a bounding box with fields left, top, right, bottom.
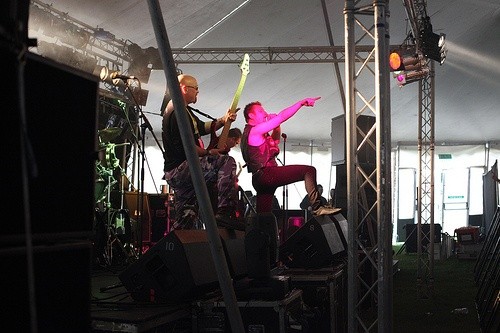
left=110, top=191, right=151, bottom=244
left=331, top=214, right=348, bottom=260
left=0, top=42, right=98, bottom=242
left=329, top=114, right=377, bottom=166
left=218, top=227, right=249, bottom=277
left=282, top=214, right=344, bottom=268
left=0, top=242, right=95, bottom=333
left=118, top=229, right=225, bottom=309
left=336, top=163, right=376, bottom=249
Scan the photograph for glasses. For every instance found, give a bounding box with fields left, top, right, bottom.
left=180, top=85, right=198, bottom=91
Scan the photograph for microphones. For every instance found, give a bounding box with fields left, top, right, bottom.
left=112, top=75, right=137, bottom=80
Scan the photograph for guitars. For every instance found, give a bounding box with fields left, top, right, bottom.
left=205, top=52, right=253, bottom=156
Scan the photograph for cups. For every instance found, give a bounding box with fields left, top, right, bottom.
left=160, top=185, right=167, bottom=194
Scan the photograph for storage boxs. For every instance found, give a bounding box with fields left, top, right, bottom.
left=190, top=247, right=376, bottom=333
left=0, top=38, right=100, bottom=333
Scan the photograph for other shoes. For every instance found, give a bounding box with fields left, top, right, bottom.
left=216, top=208, right=247, bottom=224
left=312, top=206, right=342, bottom=217
left=271, top=268, right=286, bottom=277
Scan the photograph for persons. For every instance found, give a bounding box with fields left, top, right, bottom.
left=206, top=128, right=243, bottom=156
left=240, top=96, right=342, bottom=217
left=160, top=74, right=241, bottom=232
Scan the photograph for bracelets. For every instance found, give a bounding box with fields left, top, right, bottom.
left=206, top=149, right=211, bottom=155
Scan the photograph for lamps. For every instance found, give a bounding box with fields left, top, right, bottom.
left=29, top=4, right=164, bottom=106
left=389, top=31, right=448, bottom=89
